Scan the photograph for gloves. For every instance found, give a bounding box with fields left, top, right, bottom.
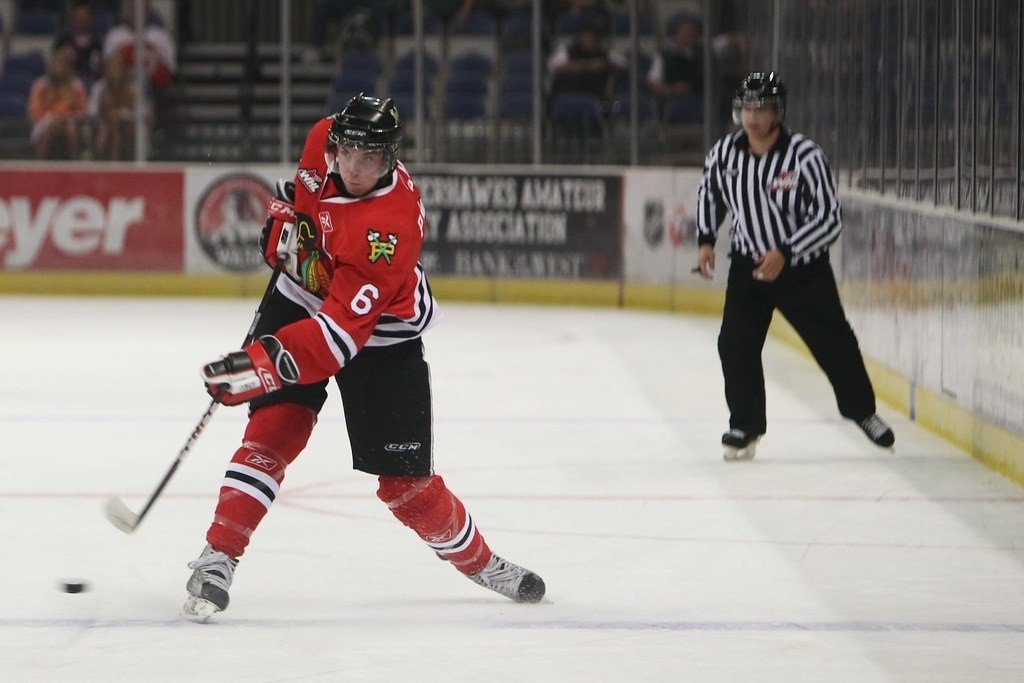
left=201, top=334, right=300, bottom=406
left=258, top=178, right=295, bottom=273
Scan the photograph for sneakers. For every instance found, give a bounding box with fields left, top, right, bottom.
left=467, top=551, right=545, bottom=604
left=722, top=429, right=762, bottom=462
left=180, top=543, right=239, bottom=623
left=856, top=414, right=895, bottom=453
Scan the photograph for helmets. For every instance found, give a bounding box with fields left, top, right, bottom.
left=325, top=92, right=404, bottom=178
left=732, top=72, right=786, bottom=131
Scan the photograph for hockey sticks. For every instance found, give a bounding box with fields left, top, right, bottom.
left=99, top=257, right=282, bottom=536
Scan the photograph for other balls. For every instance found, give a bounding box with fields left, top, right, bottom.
left=61, top=583, right=83, bottom=593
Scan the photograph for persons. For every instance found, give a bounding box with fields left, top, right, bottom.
left=695, top=70, right=897, bottom=462
left=0, top=1, right=746, bottom=163
left=179, top=95, right=546, bottom=625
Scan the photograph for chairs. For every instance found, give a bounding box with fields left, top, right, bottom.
left=0, top=0, right=168, bottom=160
left=330, top=47, right=657, bottom=163
